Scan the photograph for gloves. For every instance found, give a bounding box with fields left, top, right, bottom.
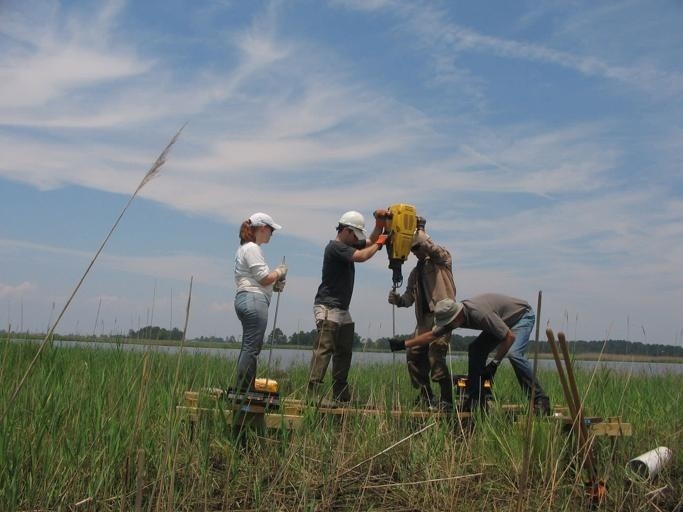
left=387, top=336, right=408, bottom=353
left=375, top=232, right=390, bottom=250
left=273, top=264, right=290, bottom=293
left=387, top=291, right=401, bottom=304
left=480, top=361, right=499, bottom=380
left=373, top=209, right=388, bottom=228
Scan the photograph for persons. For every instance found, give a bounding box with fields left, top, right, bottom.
left=231, top=212, right=289, bottom=394
left=388, top=292, right=551, bottom=419
left=385, top=226, right=458, bottom=413
left=305, top=208, right=393, bottom=409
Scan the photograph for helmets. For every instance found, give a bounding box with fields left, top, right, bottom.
left=337, top=210, right=368, bottom=234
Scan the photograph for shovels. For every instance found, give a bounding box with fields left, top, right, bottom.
left=546, top=327, right=610, bottom=512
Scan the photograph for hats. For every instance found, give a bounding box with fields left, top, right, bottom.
left=249, top=212, right=282, bottom=230
left=433, top=298, right=464, bottom=327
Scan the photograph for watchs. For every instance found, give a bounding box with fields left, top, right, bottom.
left=492, top=359, right=500, bottom=366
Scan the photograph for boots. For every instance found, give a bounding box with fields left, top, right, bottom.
left=411, top=378, right=452, bottom=410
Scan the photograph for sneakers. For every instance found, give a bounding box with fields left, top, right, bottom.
left=523, top=393, right=552, bottom=418
left=330, top=381, right=355, bottom=403
left=304, top=378, right=340, bottom=410
